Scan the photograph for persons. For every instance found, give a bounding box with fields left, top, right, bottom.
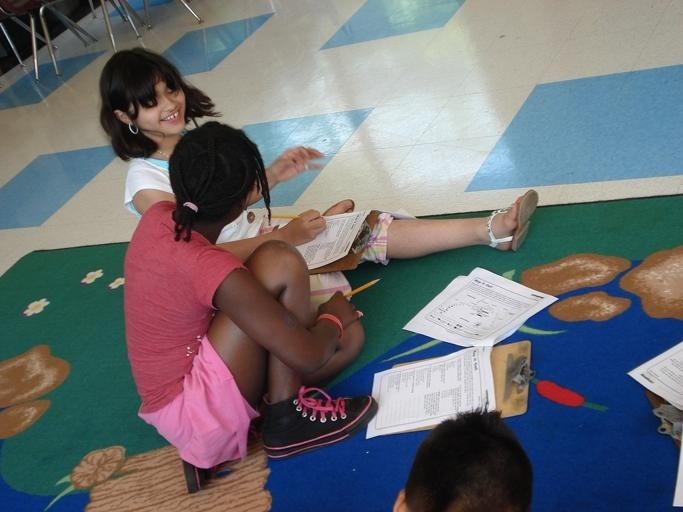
left=120, top=121, right=377, bottom=473
left=394, top=408, right=532, bottom=511
left=98, top=48, right=537, bottom=320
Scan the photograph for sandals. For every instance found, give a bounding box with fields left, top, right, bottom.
left=487, top=190, right=538, bottom=253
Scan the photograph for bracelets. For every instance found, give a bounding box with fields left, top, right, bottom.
left=313, top=312, right=344, bottom=341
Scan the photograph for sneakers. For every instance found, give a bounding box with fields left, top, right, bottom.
left=178, top=459, right=216, bottom=494
left=261, top=394, right=378, bottom=458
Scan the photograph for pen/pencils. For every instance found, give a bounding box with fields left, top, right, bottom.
left=264, top=214, right=299, bottom=219
left=343, top=278, right=382, bottom=298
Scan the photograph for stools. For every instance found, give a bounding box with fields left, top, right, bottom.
left=0, top=2, right=209, bottom=85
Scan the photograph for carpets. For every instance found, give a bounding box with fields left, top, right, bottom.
left=1, top=188, right=683, bottom=511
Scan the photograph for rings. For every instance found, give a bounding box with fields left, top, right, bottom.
left=303, top=164, right=310, bottom=171
